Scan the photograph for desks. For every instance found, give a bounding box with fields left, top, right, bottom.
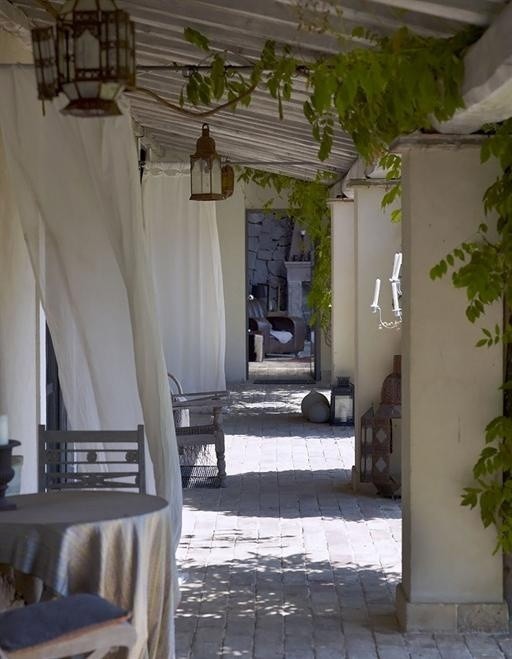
left=1, top=490, right=178, bottom=659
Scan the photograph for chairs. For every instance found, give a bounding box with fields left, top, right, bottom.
left=247, top=298, right=305, bottom=358
left=171, top=391, right=231, bottom=489
left=0, top=422, right=147, bottom=659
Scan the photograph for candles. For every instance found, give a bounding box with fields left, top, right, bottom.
left=1, top=411, right=10, bottom=444
left=372, top=251, right=403, bottom=309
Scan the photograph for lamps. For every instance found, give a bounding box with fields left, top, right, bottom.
left=33, top=0, right=136, bottom=118
left=189, top=115, right=224, bottom=200
